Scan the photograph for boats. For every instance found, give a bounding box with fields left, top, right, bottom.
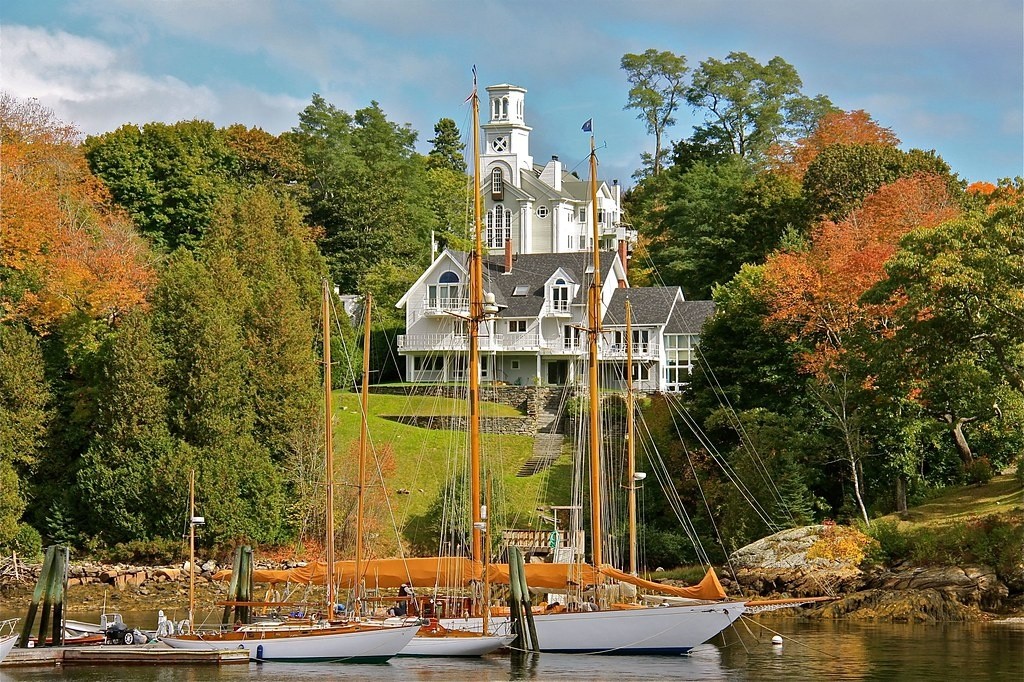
left=0, top=617, right=20, bottom=663
left=61, top=618, right=157, bottom=640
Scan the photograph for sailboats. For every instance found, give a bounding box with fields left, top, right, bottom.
left=385, top=118, right=842, bottom=654
left=160, top=470, right=430, bottom=664
left=237, top=65, right=518, bottom=658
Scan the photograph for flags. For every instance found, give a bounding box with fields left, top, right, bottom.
left=581, top=117, right=591, bottom=132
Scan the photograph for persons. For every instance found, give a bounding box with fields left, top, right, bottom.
left=399, top=581, right=414, bottom=614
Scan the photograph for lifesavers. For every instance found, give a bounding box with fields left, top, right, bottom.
left=160, top=619, right=175, bottom=637
left=177, top=618, right=194, bottom=636
